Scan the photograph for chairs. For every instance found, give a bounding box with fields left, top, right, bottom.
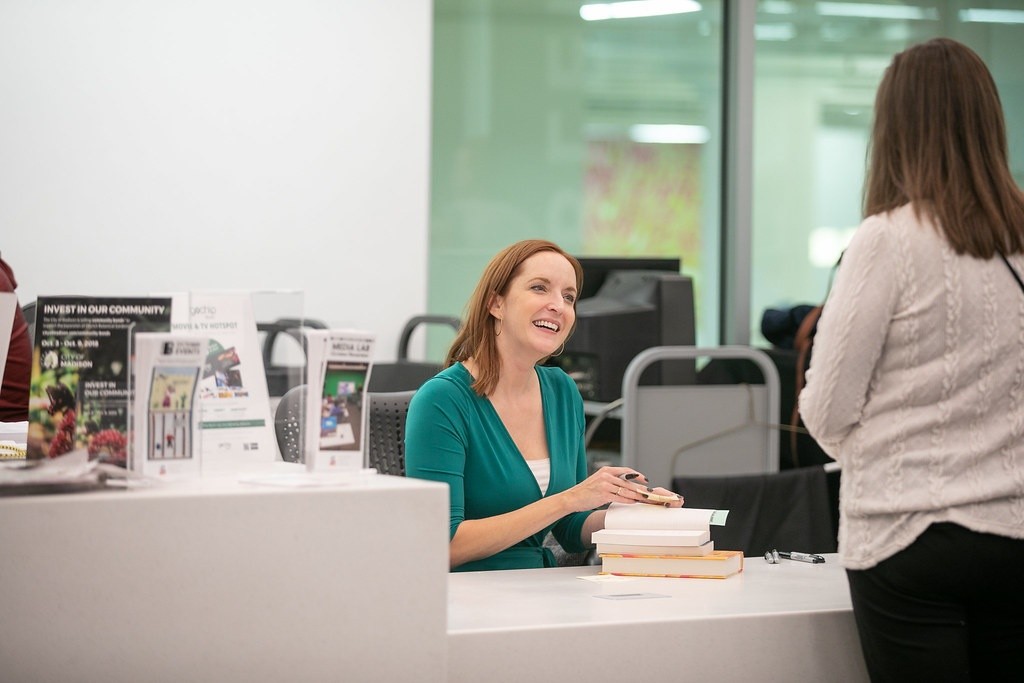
left=276, top=384, right=416, bottom=477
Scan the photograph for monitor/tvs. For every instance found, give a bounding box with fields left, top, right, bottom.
left=551, top=268, right=696, bottom=401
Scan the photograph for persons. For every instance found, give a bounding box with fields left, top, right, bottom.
left=323, top=394, right=350, bottom=419
left=404, top=238, right=685, bottom=571
left=797, top=34, right=1024, bottom=682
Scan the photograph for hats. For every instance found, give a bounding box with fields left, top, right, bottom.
left=760, top=306, right=822, bottom=348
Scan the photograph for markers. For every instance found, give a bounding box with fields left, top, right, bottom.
left=618, top=472, right=641, bottom=481
left=764, top=548, right=826, bottom=564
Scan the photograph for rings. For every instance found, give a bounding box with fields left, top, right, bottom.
left=616, top=487, right=621, bottom=495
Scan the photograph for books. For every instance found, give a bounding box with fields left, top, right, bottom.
left=592, top=501, right=744, bottom=580
